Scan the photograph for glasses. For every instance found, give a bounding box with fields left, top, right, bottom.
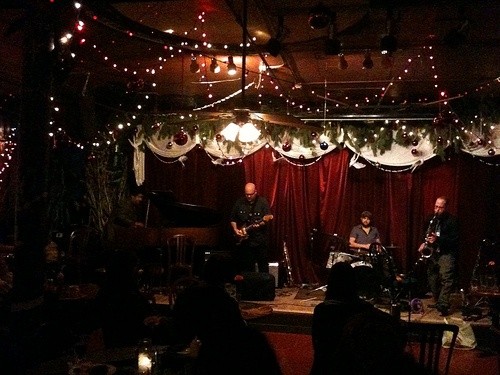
left=244, top=190, right=255, bottom=196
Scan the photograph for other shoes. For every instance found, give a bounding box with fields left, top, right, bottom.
left=427, top=304, right=437, bottom=308
left=437, top=305, right=448, bottom=316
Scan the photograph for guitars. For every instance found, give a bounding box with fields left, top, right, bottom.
left=233, top=214, right=274, bottom=246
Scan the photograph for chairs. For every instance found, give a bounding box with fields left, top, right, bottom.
left=408, top=323, right=459, bottom=375
left=167, top=234, right=195, bottom=275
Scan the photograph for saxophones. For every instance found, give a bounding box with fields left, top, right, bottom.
left=420, top=212, right=439, bottom=258
left=281, top=234, right=294, bottom=287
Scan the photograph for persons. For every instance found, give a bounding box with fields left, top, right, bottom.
left=349, top=210, right=381, bottom=252
left=228, top=183, right=273, bottom=274
left=105, top=186, right=145, bottom=240
left=418, top=196, right=465, bottom=312
left=311, top=262, right=373, bottom=375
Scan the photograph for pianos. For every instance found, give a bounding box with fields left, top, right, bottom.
left=124, top=200, right=223, bottom=248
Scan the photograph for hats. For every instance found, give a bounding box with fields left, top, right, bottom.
left=360, top=211, right=372, bottom=217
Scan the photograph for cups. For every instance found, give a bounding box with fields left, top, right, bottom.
left=68, top=285, right=80, bottom=298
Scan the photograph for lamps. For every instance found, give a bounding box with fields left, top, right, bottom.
left=191, top=56, right=237, bottom=75
left=337, top=36, right=389, bottom=70
left=222, top=0, right=259, bottom=142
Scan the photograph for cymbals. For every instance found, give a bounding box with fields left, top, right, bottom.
left=386, top=245, right=401, bottom=248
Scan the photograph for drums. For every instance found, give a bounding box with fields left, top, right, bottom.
left=334, top=252, right=352, bottom=264
left=326, top=251, right=339, bottom=268
left=350, top=253, right=380, bottom=300
